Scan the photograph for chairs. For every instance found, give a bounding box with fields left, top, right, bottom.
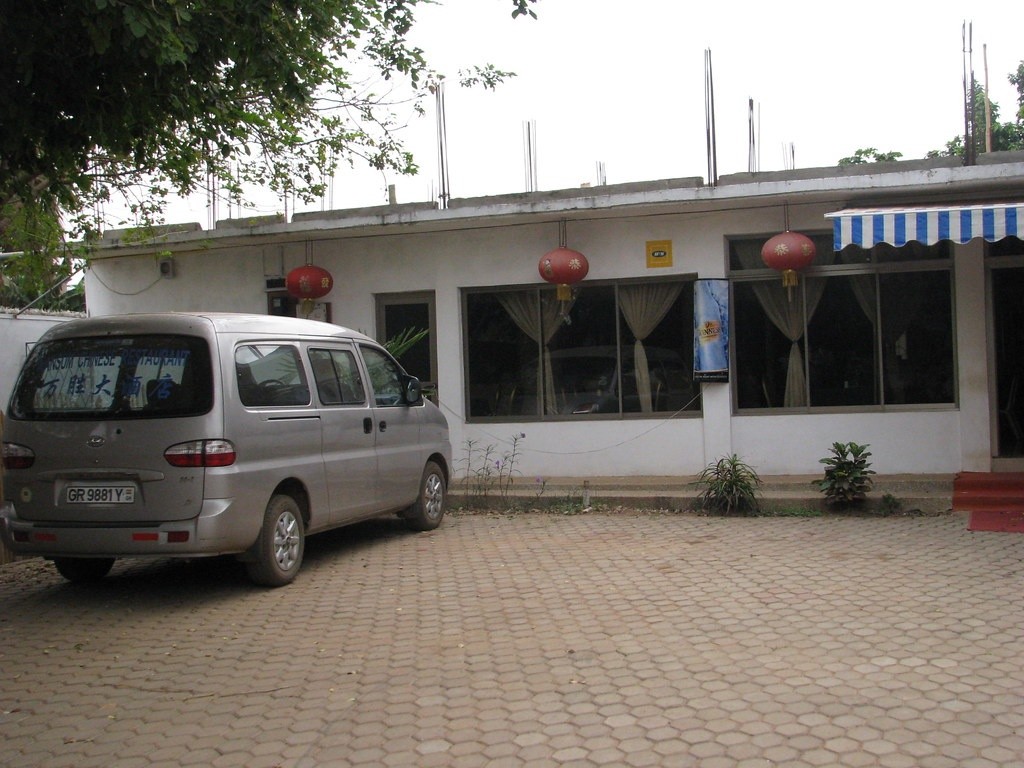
left=1000, top=375, right=1023, bottom=444
left=483, top=381, right=524, bottom=416
left=147, top=377, right=180, bottom=414
left=761, top=375, right=775, bottom=404
left=307, top=353, right=341, bottom=401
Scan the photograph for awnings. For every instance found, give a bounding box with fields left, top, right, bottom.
left=825, top=202, right=1024, bottom=251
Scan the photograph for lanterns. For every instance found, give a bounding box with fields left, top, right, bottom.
left=286, top=264, right=333, bottom=314
left=761, top=231, right=816, bottom=286
left=539, top=246, right=588, bottom=300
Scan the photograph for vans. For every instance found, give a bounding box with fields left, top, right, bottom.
left=498, top=348, right=696, bottom=411
left=1, top=312, right=455, bottom=588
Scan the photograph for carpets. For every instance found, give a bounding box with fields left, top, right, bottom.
left=954, top=470, right=1024, bottom=511
left=966, top=510, right=1024, bottom=535
